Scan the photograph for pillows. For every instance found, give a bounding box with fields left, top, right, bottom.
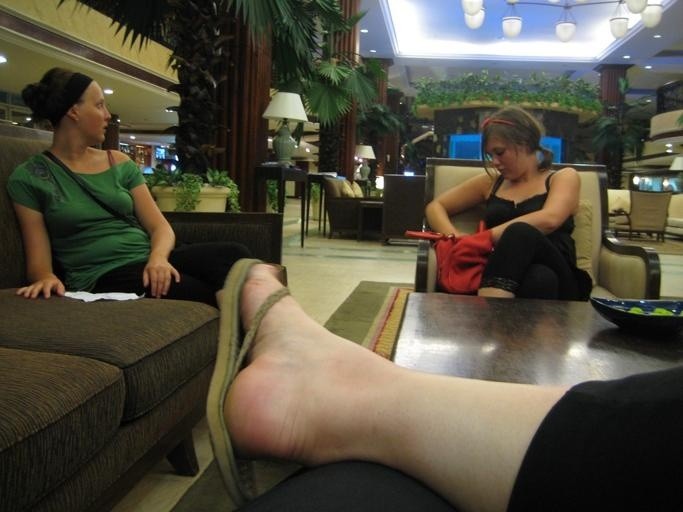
left=340, top=181, right=355, bottom=199
left=350, top=180, right=363, bottom=198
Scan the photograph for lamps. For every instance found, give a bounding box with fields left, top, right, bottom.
left=354, top=143, right=376, bottom=179
left=457, top=0, right=665, bottom=49
left=261, top=90, right=308, bottom=160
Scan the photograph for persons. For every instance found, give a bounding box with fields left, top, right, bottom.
left=206, top=259, right=682, bottom=511
left=424, top=105, right=592, bottom=299
left=6, top=66, right=256, bottom=477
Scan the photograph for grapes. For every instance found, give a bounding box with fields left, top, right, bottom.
left=629, top=307, right=683, bottom=316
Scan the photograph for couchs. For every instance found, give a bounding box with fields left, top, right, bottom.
left=0, top=121, right=283, bottom=511
left=606, top=186, right=682, bottom=237
left=412, top=156, right=663, bottom=296
left=320, top=176, right=383, bottom=240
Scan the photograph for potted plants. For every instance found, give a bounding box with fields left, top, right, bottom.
left=141, top=167, right=244, bottom=213
left=309, top=184, right=328, bottom=221
left=265, top=181, right=287, bottom=214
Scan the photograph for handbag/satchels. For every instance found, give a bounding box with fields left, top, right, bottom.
left=404, top=219, right=493, bottom=294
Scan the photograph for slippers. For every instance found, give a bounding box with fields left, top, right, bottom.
left=206, top=257, right=291, bottom=507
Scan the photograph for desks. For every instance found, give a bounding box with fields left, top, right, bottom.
left=305, top=170, right=342, bottom=239
left=168, top=274, right=682, bottom=511
left=250, top=163, right=309, bottom=248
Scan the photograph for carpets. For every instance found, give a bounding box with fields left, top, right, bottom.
left=162, top=280, right=420, bottom=510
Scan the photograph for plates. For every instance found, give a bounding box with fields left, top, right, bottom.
left=588, top=294, right=682, bottom=331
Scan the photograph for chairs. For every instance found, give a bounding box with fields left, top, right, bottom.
left=614, top=189, right=673, bottom=243
